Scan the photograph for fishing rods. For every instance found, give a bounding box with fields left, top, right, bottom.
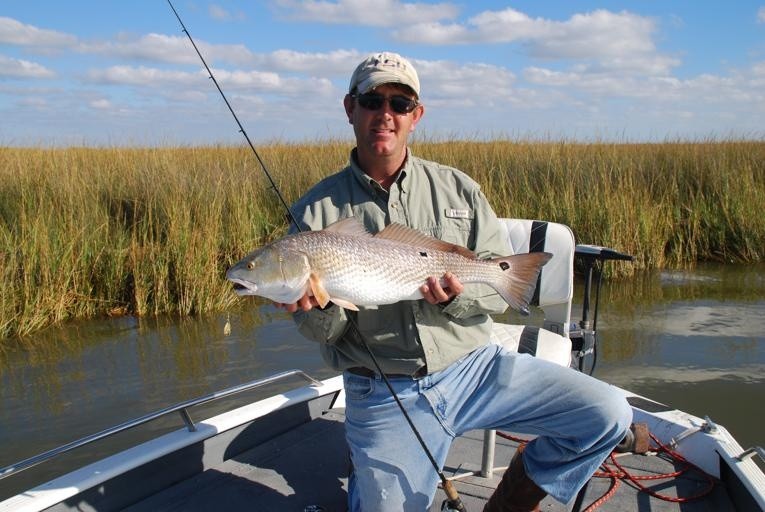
left=168, top=3, right=469, bottom=511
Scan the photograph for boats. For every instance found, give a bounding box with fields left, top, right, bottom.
left=0, top=218, right=765, bottom=511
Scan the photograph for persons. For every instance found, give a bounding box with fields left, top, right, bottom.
left=271, top=51, right=634, bottom=512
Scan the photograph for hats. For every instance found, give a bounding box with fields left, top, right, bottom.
left=349, top=53, right=420, bottom=96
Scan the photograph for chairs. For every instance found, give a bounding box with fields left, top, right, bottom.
left=480, top=215, right=576, bottom=483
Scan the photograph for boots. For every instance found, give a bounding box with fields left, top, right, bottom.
left=482, top=447, right=547, bottom=512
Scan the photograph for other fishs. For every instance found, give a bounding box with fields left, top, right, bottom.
left=224, top=215, right=553, bottom=319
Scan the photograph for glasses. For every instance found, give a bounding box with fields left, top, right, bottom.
left=352, top=90, right=419, bottom=113
